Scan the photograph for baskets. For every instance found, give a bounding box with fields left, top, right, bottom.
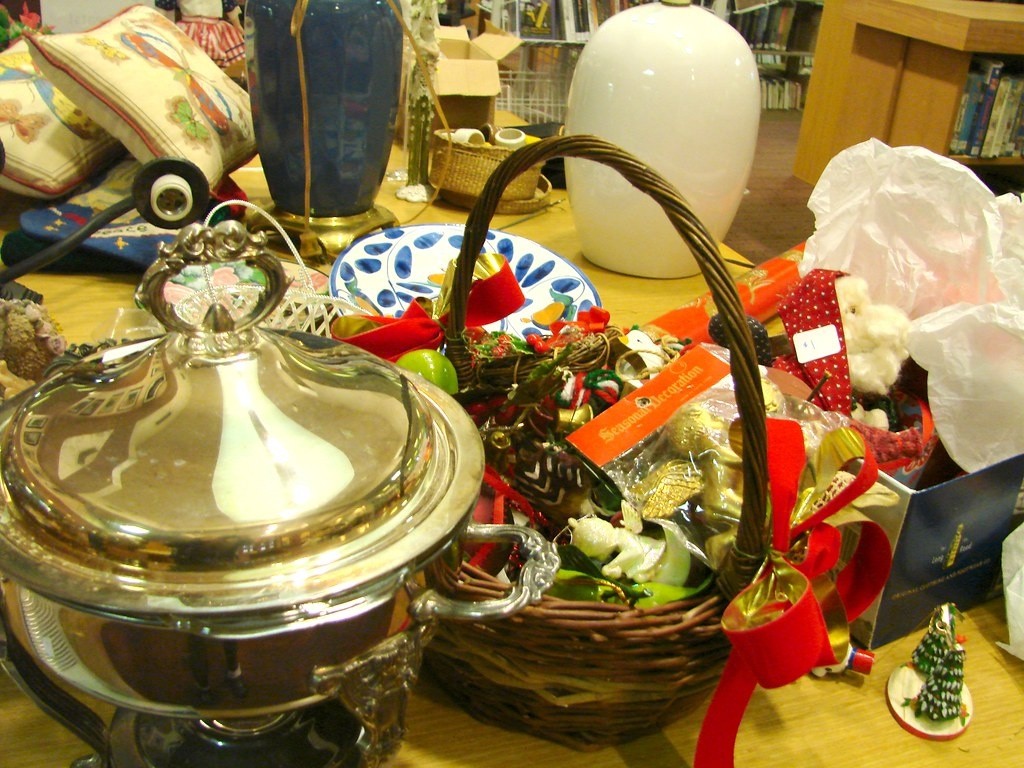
left=430, top=129, right=545, bottom=199
left=424, top=136, right=823, bottom=753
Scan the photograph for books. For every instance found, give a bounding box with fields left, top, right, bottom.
left=727, top=0, right=813, bottom=110
left=511, top=0, right=628, bottom=41
left=949, top=56, right=1024, bottom=160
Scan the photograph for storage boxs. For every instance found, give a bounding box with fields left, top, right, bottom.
left=397, top=20, right=524, bottom=148
left=841, top=453, right=1024, bottom=651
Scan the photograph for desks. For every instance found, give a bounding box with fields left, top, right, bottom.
left=1, top=150, right=1023, bottom=768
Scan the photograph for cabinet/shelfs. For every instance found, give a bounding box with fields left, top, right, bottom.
left=793, top=0, right=1024, bottom=183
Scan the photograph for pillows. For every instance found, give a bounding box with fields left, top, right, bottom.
left=0, top=38, right=127, bottom=202
left=21, top=4, right=260, bottom=196
